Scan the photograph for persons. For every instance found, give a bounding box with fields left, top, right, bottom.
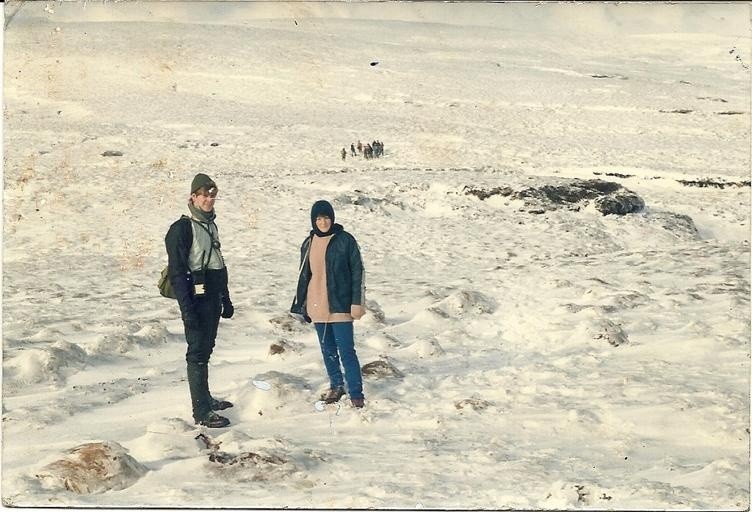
left=350, top=142, right=356, bottom=157
left=341, top=147, right=347, bottom=161
left=164, top=172, right=237, bottom=427
left=288, top=199, right=369, bottom=412
left=357, top=138, right=385, bottom=160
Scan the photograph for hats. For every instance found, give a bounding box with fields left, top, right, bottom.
left=191, top=173, right=214, bottom=193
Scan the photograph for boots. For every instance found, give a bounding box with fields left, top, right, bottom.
left=187, top=362, right=233, bottom=427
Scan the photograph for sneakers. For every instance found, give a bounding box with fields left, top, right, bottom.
left=323, top=386, right=344, bottom=403
left=351, top=396, right=364, bottom=409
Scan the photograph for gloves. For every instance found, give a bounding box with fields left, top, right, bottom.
left=219, top=297, right=234, bottom=318
left=350, top=303, right=365, bottom=319
left=179, top=303, right=200, bottom=330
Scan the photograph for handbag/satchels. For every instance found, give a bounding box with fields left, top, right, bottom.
left=158, top=265, right=179, bottom=300
